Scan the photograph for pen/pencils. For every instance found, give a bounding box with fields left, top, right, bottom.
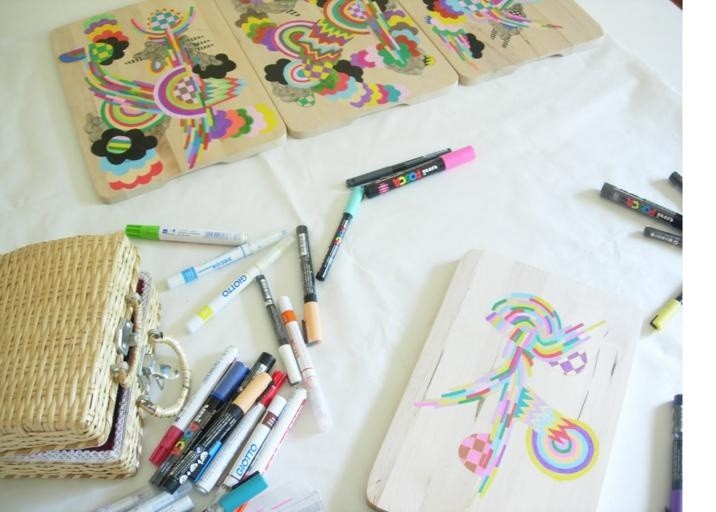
left=669, top=171, right=683, bottom=189
left=317, top=185, right=365, bottom=281
left=650, top=292, right=682, bottom=329
left=347, top=146, right=476, bottom=198
left=93, top=470, right=270, bottom=512
left=167, top=230, right=284, bottom=289
left=125, top=224, right=247, bottom=246
left=186, top=235, right=296, bottom=332
left=601, top=182, right=682, bottom=247
left=148, top=345, right=309, bottom=494
left=256, top=227, right=320, bottom=389
left=665, top=394, right=683, bottom=512
